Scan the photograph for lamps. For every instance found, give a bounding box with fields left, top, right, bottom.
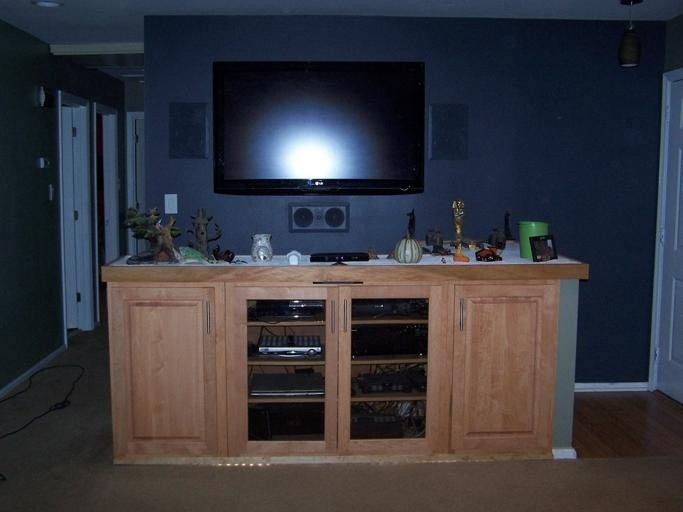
left=619, top=0, right=643, bottom=68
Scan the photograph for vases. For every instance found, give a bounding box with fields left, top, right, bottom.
left=251, top=234, right=273, bottom=261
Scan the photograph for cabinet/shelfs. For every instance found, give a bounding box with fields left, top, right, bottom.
left=226, top=283, right=442, bottom=464
left=107, top=283, right=228, bottom=466
left=442, top=280, right=561, bottom=463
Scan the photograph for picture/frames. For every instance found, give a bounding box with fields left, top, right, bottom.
left=529, top=235, right=557, bottom=262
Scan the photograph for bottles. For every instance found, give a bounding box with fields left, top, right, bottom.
left=425, top=228, right=443, bottom=247
left=251, top=233, right=273, bottom=262
left=489, top=228, right=507, bottom=250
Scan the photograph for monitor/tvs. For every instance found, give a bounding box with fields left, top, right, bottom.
left=212, top=60, right=426, bottom=195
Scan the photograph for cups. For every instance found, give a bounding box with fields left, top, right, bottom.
left=518, top=221, right=549, bottom=259
